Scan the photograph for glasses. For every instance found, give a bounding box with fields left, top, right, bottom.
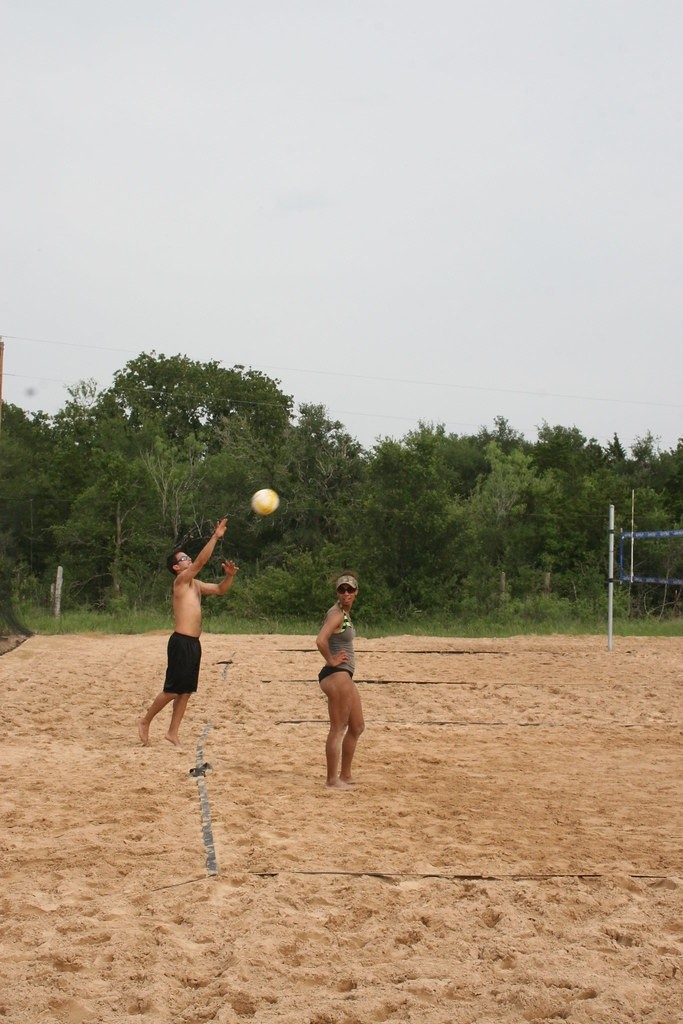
left=337, top=585, right=356, bottom=594
left=173, top=555, right=190, bottom=564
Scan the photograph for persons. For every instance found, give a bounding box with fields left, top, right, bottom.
left=138, top=519, right=239, bottom=747
left=316, top=571, right=366, bottom=791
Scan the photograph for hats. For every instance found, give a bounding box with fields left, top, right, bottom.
left=336, top=575, right=359, bottom=589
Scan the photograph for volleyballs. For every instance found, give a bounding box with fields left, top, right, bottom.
left=251, top=489, right=279, bottom=515
